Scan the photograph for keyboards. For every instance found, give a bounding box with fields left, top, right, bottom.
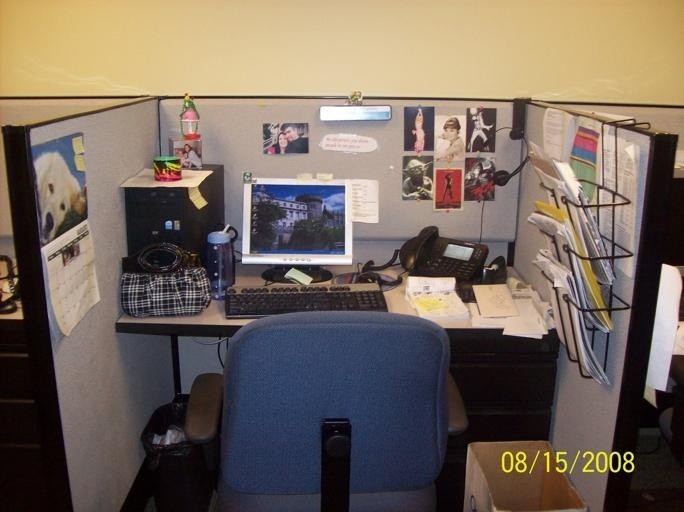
left=225, top=284, right=388, bottom=319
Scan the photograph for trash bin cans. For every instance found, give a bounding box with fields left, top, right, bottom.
left=140, top=397, right=197, bottom=512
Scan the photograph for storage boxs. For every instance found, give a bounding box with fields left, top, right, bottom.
left=464, top=441, right=590, bottom=512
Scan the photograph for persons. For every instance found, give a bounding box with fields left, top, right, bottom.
left=438, top=173, right=455, bottom=203
left=402, top=159, right=431, bottom=200
left=267, top=133, right=288, bottom=155
left=280, top=124, right=308, bottom=153
left=464, top=161, right=495, bottom=192
left=468, top=112, right=494, bottom=153
left=183, top=143, right=201, bottom=168
left=412, top=109, right=425, bottom=152
left=436, top=117, right=464, bottom=161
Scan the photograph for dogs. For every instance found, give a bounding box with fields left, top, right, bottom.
left=34, top=151, right=86, bottom=245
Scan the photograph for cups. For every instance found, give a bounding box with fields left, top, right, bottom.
left=151, top=156, right=183, bottom=183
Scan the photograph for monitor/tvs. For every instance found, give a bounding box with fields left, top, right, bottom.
left=242, top=178, right=352, bottom=284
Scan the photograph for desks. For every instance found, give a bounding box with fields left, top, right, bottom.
left=115, top=265, right=561, bottom=394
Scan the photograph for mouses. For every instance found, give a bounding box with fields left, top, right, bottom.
left=359, top=272, right=382, bottom=287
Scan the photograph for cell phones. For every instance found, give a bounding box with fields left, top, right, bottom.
left=460, top=279, right=475, bottom=303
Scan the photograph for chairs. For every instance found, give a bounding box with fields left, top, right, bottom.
left=184, top=311, right=468, bottom=512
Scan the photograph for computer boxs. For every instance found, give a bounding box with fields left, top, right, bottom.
left=125, top=164, right=224, bottom=267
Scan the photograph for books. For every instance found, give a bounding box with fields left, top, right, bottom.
left=471, top=283, right=518, bottom=319
left=526, top=138, right=615, bottom=386
left=404, top=273, right=469, bottom=325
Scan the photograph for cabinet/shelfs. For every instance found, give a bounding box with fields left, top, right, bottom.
left=0, top=321, right=39, bottom=512
left=439, top=331, right=553, bottom=504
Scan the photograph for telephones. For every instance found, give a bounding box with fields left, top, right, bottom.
left=401, top=226, right=489, bottom=285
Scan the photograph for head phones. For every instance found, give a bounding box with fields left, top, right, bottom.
left=482, top=127, right=530, bottom=186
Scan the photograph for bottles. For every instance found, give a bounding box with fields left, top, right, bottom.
left=204, top=228, right=236, bottom=300
left=178, top=95, right=199, bottom=139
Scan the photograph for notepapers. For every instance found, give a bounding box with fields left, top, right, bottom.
left=286, top=269, right=314, bottom=287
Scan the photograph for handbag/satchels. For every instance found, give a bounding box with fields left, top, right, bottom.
left=120, top=241, right=213, bottom=319
